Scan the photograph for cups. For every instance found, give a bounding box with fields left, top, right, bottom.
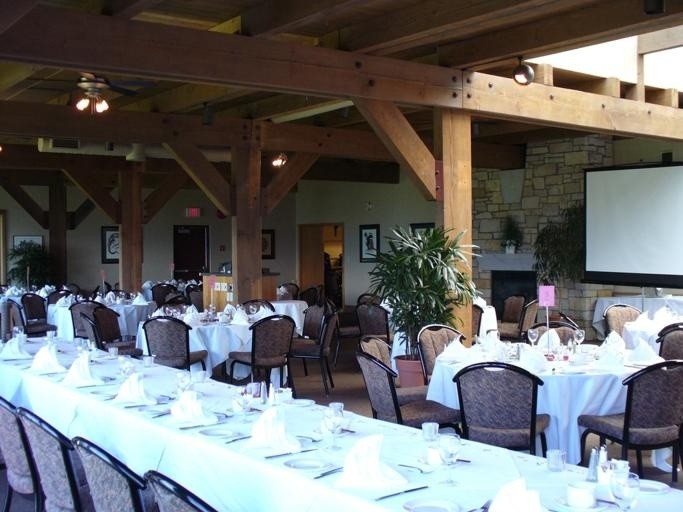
left=109, top=347, right=118, bottom=359
left=422, top=422, right=439, bottom=441
left=565, top=481, right=596, bottom=508
left=546, top=449, right=566, bottom=473
left=165, top=306, right=173, bottom=316
left=198, top=371, right=209, bottom=383
left=13, top=326, right=25, bottom=347
left=143, top=357, right=153, bottom=368
left=175, top=371, right=191, bottom=392
left=437, top=433, right=462, bottom=488
left=130, top=292, right=136, bottom=301
left=249, top=304, right=257, bottom=322
left=528, top=329, right=538, bottom=349
left=573, top=329, right=585, bottom=352
left=610, top=472, right=640, bottom=512
left=329, top=402, right=345, bottom=409
left=324, top=407, right=345, bottom=451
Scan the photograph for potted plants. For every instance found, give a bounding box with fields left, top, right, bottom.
left=498, top=299, right=539, bottom=343
left=499, top=211, right=525, bottom=255
left=365, top=224, right=484, bottom=389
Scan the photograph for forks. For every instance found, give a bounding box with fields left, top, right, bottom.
left=296, top=435, right=322, bottom=443
left=399, top=464, right=432, bottom=474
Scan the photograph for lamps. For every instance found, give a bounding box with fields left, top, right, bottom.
left=513, top=57, right=536, bottom=86
left=271, top=153, right=289, bottom=169
left=76, top=76, right=109, bottom=117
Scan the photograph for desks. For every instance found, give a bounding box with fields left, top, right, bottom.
left=426, top=343, right=680, bottom=473
left=259, top=300, right=309, bottom=336
left=136, top=312, right=288, bottom=389
left=145, top=283, right=203, bottom=301
left=47, top=298, right=157, bottom=342
left=622, top=319, right=683, bottom=357
left=592, top=295, right=683, bottom=339
left=0, top=290, right=66, bottom=307
left=380, top=296, right=499, bottom=374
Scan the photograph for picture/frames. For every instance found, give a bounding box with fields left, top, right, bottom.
left=262, top=229, right=277, bottom=261
left=357, top=223, right=381, bottom=264
left=12, top=235, right=46, bottom=252
left=409, top=223, right=437, bottom=242
left=100, top=225, right=144, bottom=265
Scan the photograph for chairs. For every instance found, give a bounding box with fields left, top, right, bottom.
left=20, top=293, right=57, bottom=338
left=142, top=315, right=208, bottom=372
left=155, top=295, right=192, bottom=312
left=356, top=292, right=382, bottom=340
left=189, top=289, right=204, bottom=313
left=7, top=299, right=47, bottom=336
left=150, top=284, right=177, bottom=307
left=91, top=307, right=136, bottom=352
left=495, top=294, right=526, bottom=332
left=359, top=335, right=429, bottom=407
left=79, top=311, right=140, bottom=360
left=0, top=396, right=43, bottom=512
left=355, top=302, right=394, bottom=355
left=228, top=314, right=296, bottom=397
left=104, top=282, right=112, bottom=297
left=102, top=290, right=130, bottom=300
left=68, top=299, right=108, bottom=342
left=145, top=471, right=218, bottom=512
left=186, top=282, right=203, bottom=306
left=354, top=351, right=464, bottom=439
left=86, top=285, right=100, bottom=301
left=17, top=407, right=75, bottom=512
left=46, top=289, right=70, bottom=307
left=452, top=363, right=551, bottom=459
left=278, top=282, right=301, bottom=300
left=115, top=282, right=120, bottom=290
left=472, top=303, right=484, bottom=346
left=0, top=285, right=8, bottom=294
left=289, top=310, right=340, bottom=394
left=67, top=282, right=81, bottom=294
left=73, top=436, right=146, bottom=512
left=559, top=311, right=605, bottom=346
left=526, top=321, right=578, bottom=346
left=603, top=303, right=642, bottom=339
left=416, top=323, right=467, bottom=385
left=577, top=362, right=683, bottom=484
left=288, top=301, right=331, bottom=381
left=239, top=298, right=276, bottom=315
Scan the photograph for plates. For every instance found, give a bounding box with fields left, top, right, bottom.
left=545, top=496, right=621, bottom=512
left=638, top=479, right=671, bottom=495
left=90, top=390, right=118, bottom=396
left=402, top=499, right=462, bottom=512
left=199, top=428, right=234, bottom=437
left=138, top=404, right=169, bottom=416
left=283, top=457, right=331, bottom=470
left=282, top=399, right=316, bottom=407
